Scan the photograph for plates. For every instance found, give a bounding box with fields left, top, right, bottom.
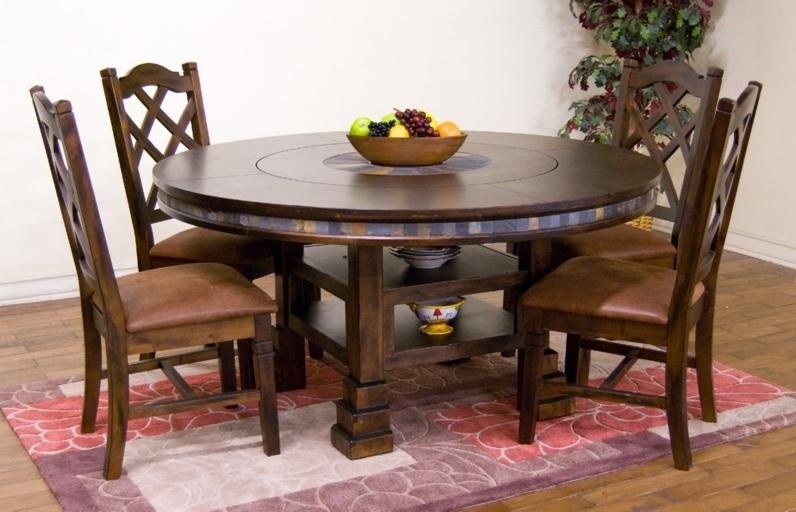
left=388, top=246, right=462, bottom=268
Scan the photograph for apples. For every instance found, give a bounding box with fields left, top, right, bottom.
left=381, top=113, right=400, bottom=126
left=350, top=117, right=373, bottom=137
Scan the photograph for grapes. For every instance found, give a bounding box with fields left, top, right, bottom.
left=368, top=120, right=396, bottom=137
left=394, top=105, right=439, bottom=137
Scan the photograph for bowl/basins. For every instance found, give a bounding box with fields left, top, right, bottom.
left=344, top=133, right=467, bottom=167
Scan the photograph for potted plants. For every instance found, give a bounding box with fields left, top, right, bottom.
left=554, top=0, right=715, bottom=236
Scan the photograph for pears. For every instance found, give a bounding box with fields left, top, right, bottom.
left=418, top=109, right=437, bottom=132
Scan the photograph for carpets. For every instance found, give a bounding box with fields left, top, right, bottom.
left=2, top=308, right=796, bottom=511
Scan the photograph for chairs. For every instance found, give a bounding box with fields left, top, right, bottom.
left=500, top=58, right=724, bottom=383
left=30, top=85, right=280, bottom=479
left=517, top=81, right=762, bottom=469
left=99, top=63, right=315, bottom=408
